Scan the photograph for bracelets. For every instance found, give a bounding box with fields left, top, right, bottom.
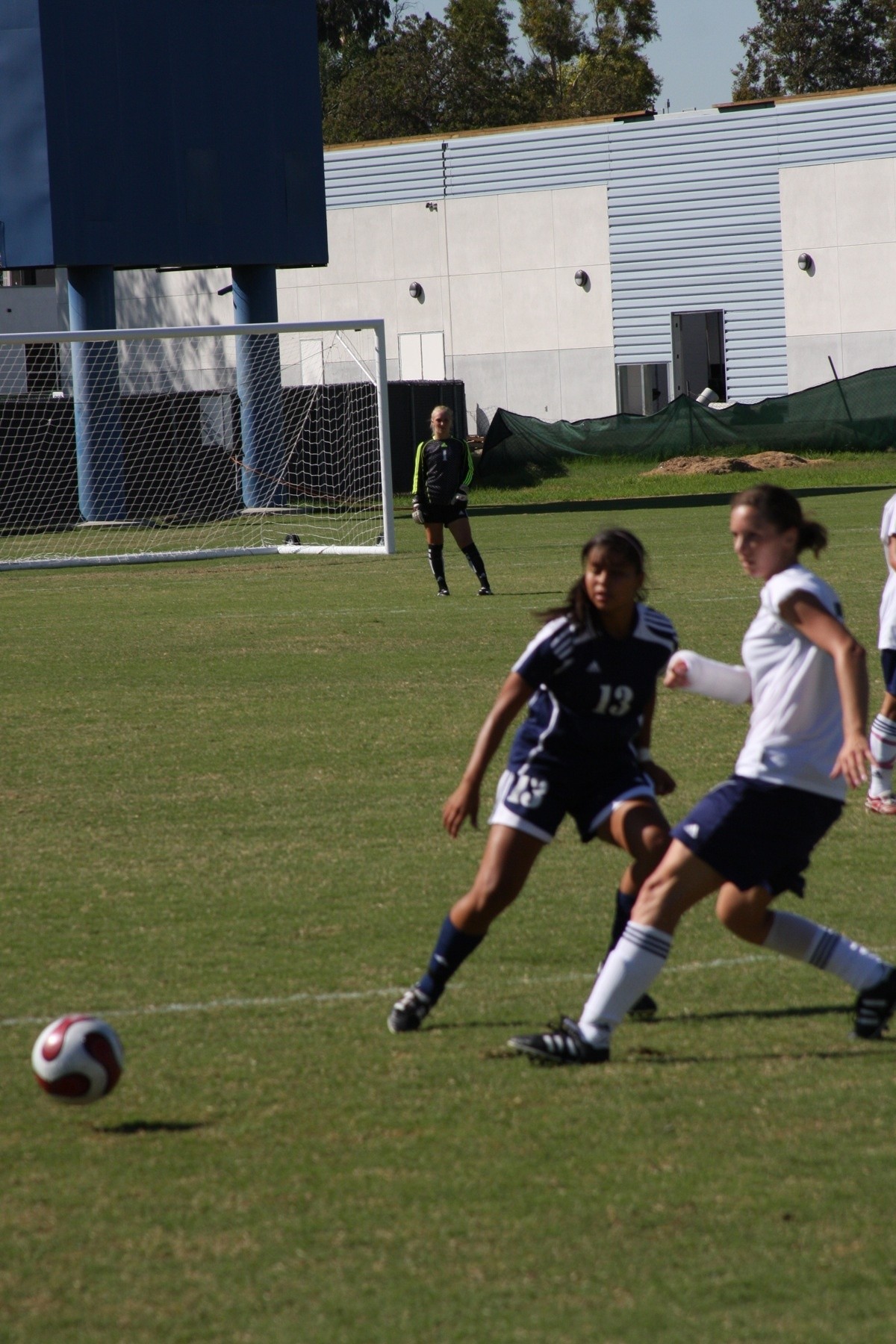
left=639, top=746, right=654, bottom=762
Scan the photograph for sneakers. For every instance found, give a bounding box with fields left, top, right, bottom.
left=387, top=982, right=442, bottom=1035
left=598, top=959, right=657, bottom=1017
left=506, top=1016, right=610, bottom=1064
left=847, top=966, right=896, bottom=1038
left=864, top=787, right=896, bottom=815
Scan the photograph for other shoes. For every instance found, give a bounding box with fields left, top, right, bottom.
left=478, top=586, right=491, bottom=595
left=437, top=588, right=449, bottom=597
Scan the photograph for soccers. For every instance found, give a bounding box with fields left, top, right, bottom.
left=31, top=1011, right=125, bottom=1105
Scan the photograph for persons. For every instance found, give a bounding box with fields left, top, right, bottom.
left=506, top=484, right=895, bottom=1065
left=412, top=406, right=493, bottom=594
left=385, top=527, right=678, bottom=1036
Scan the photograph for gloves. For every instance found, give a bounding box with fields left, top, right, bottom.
left=450, top=494, right=468, bottom=514
left=412, top=502, right=424, bottom=523
